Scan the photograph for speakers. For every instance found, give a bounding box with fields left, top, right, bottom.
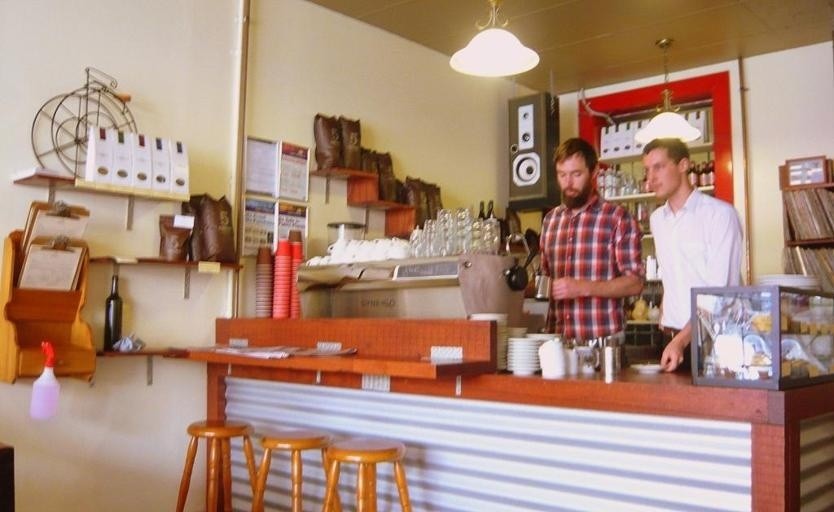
left=509, top=91, right=561, bottom=212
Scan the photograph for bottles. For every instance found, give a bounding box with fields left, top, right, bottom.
left=103, top=272, right=124, bottom=349
left=598, top=158, right=719, bottom=196
left=599, top=334, right=622, bottom=376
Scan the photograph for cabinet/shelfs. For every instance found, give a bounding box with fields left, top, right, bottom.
left=307, top=166, right=420, bottom=240
left=599, top=179, right=717, bottom=325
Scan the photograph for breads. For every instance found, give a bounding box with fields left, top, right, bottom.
left=780, top=314, right=833, bottom=336
left=781, top=356, right=820, bottom=378
left=754, top=315, right=771, bottom=332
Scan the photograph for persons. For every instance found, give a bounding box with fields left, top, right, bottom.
left=525, top=135, right=646, bottom=369
left=642, top=137, right=746, bottom=372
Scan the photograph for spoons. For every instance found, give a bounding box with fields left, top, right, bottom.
left=739, top=334, right=829, bottom=378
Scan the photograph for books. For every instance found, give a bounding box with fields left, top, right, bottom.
left=785, top=188, right=834, bottom=299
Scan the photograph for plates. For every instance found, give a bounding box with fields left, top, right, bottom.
left=759, top=272, right=821, bottom=293
left=467, top=312, right=562, bottom=377
left=631, top=362, right=663, bottom=374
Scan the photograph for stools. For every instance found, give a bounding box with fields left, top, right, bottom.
left=175, top=419, right=414, bottom=512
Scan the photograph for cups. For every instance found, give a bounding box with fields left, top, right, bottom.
left=306, top=238, right=412, bottom=265
left=410, top=205, right=500, bottom=256
left=252, top=228, right=306, bottom=319
